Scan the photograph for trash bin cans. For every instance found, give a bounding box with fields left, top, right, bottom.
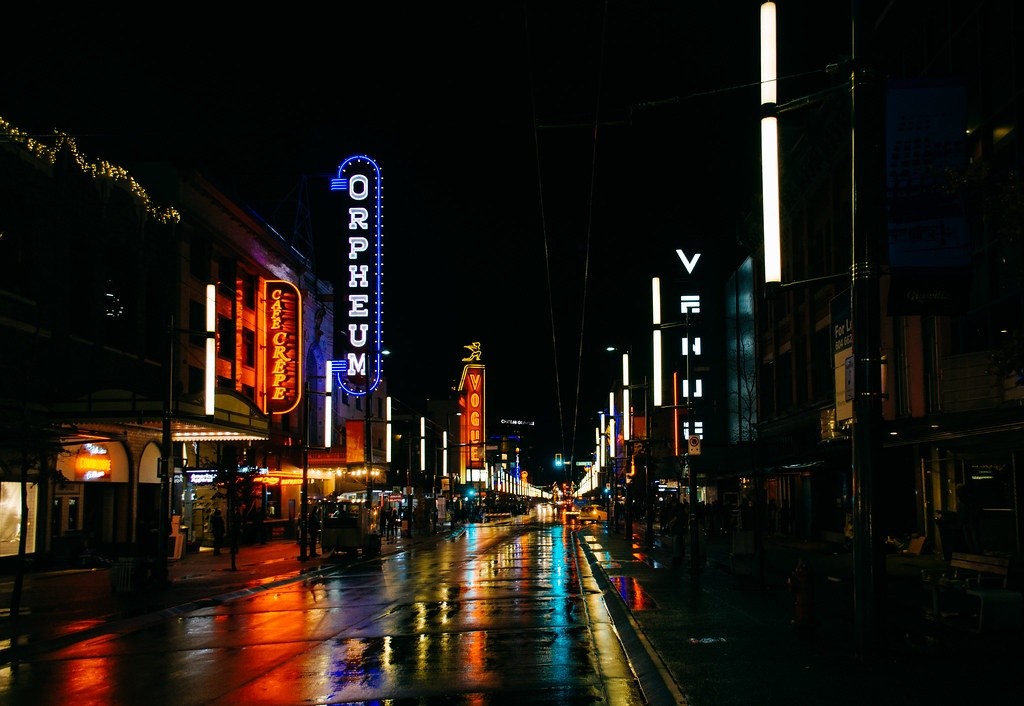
left=114, top=546, right=151, bottom=608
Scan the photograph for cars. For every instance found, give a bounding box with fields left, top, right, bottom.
left=579, top=504, right=608, bottom=523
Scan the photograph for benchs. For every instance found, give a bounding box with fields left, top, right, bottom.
left=922, top=552, right=1009, bottom=624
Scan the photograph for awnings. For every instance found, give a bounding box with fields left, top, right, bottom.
left=37, top=409, right=270, bottom=442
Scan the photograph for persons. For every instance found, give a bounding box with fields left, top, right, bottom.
left=310, top=494, right=530, bottom=557
left=210, top=509, right=224, bottom=556
left=227, top=508, right=240, bottom=554
left=614, top=493, right=731, bottom=564
